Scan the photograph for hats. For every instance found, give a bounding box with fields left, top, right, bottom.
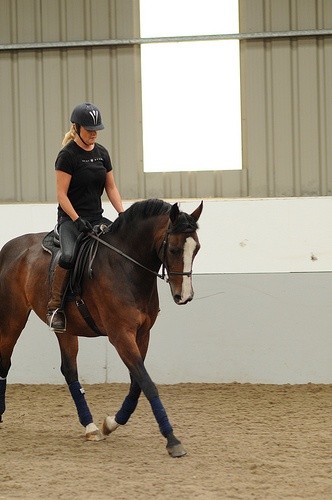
left=70, top=103, right=104, bottom=131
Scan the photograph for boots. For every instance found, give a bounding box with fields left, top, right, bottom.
left=46, top=264, right=72, bottom=330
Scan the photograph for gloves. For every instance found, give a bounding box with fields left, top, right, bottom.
left=73, top=216, right=93, bottom=235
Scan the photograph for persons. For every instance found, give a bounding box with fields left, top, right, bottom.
left=46, top=103, right=126, bottom=335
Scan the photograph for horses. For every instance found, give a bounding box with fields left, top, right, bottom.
left=0, top=198, right=204, bottom=457
